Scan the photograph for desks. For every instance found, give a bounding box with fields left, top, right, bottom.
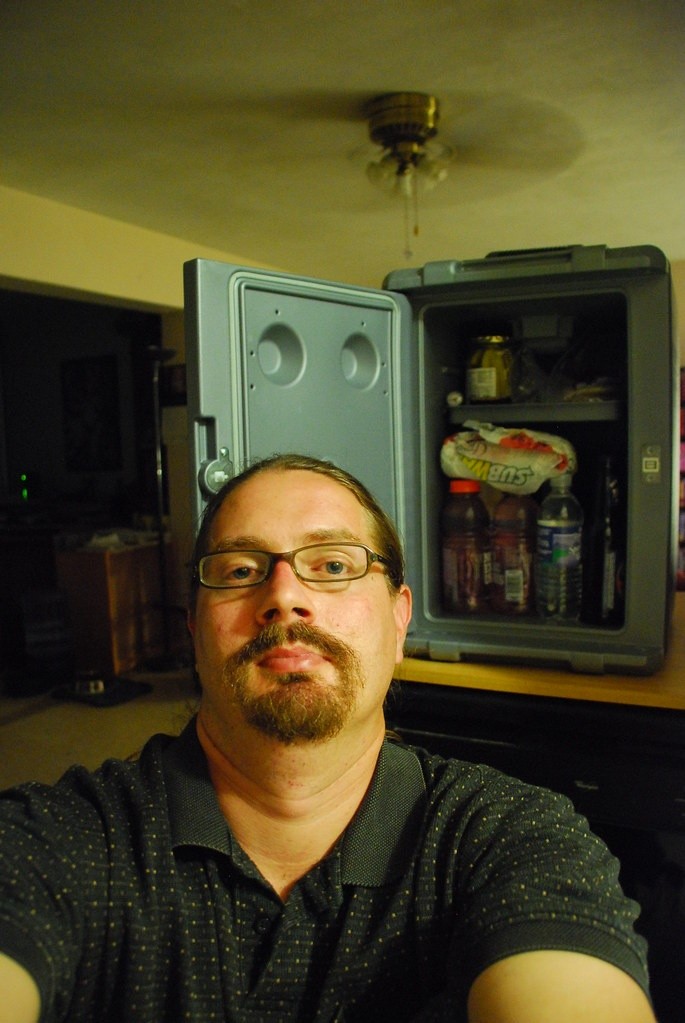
left=54, top=524, right=162, bottom=675
left=393, top=591, right=685, bottom=840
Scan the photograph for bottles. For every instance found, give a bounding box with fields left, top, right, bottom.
left=492, top=492, right=530, bottom=617
left=441, top=480, right=491, bottom=613
left=581, top=455, right=616, bottom=623
left=535, top=472, right=582, bottom=624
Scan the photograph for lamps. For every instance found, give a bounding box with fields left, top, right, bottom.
left=363, top=89, right=451, bottom=249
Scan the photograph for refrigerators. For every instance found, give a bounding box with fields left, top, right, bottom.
left=183, top=245, right=679, bottom=676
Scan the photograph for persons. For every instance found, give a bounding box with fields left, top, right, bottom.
left=0, top=453, right=660, bottom=1023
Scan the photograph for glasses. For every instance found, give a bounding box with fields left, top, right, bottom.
left=191, top=541, right=399, bottom=589
